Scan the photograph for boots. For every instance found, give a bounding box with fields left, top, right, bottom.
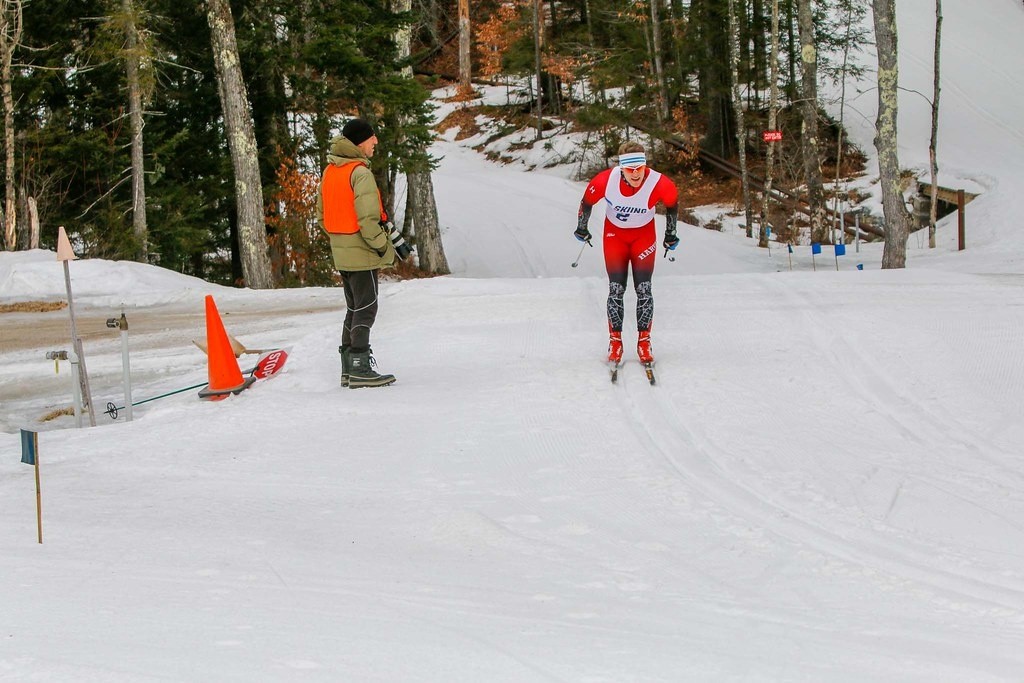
left=348, top=347, right=396, bottom=389
left=637, top=331, right=654, bottom=363
left=339, top=345, right=349, bottom=387
left=607, top=333, right=623, bottom=362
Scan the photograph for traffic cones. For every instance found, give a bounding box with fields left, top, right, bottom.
left=198, top=294, right=256, bottom=398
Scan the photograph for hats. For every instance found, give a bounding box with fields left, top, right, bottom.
left=343, top=119, right=375, bottom=146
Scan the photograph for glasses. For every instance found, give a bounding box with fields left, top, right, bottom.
left=621, top=165, right=647, bottom=174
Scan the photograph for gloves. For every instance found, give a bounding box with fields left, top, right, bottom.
left=663, top=234, right=680, bottom=250
left=372, top=246, right=387, bottom=257
left=574, top=227, right=592, bottom=242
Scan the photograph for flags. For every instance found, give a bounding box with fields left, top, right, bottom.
left=813, top=243, right=822, bottom=254
left=835, top=244, right=845, bottom=256
left=788, top=245, right=793, bottom=253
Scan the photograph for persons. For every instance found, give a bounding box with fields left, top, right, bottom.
left=317, top=120, right=417, bottom=389
left=574, top=142, right=680, bottom=368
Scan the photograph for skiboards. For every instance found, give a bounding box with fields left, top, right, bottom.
left=611, top=358, right=656, bottom=385
